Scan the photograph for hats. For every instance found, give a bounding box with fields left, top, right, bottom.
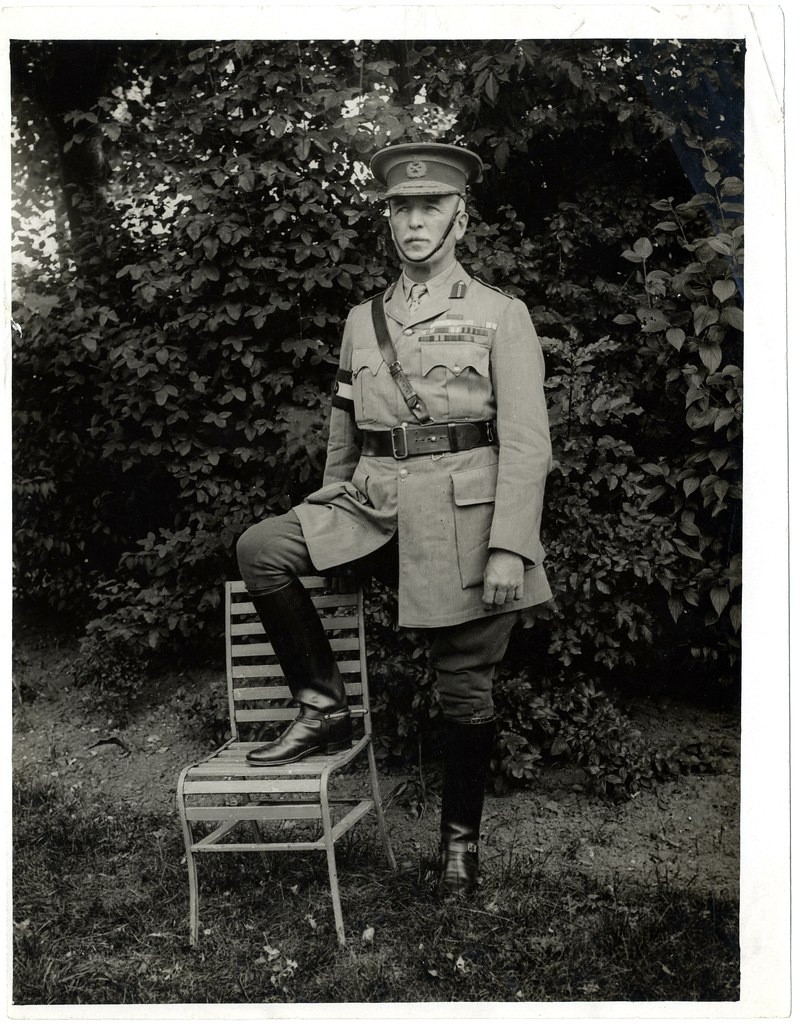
left=370, top=143, right=482, bottom=202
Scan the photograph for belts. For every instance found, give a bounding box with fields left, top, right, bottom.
left=361, top=420, right=496, bottom=461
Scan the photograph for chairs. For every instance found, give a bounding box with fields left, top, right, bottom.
left=175, top=577, right=397, bottom=949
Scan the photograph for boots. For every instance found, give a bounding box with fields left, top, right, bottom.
left=435, top=715, right=498, bottom=909
left=244, top=576, right=352, bottom=765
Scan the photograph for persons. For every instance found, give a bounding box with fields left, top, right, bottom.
left=231, top=141, right=559, bottom=909
